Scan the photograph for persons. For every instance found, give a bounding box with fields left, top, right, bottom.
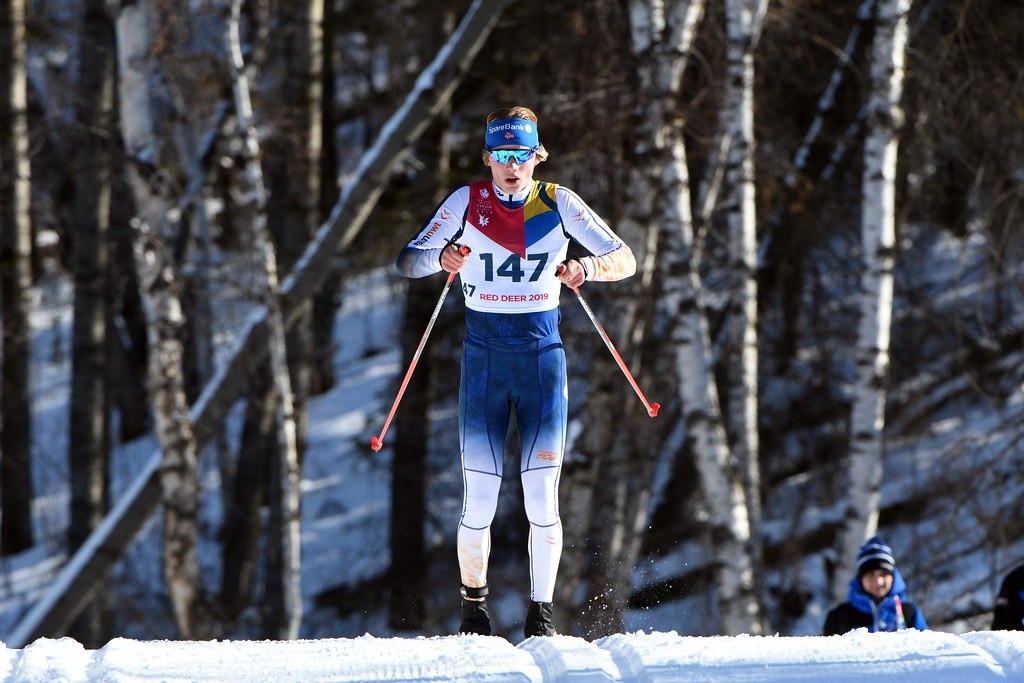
left=990, top=562, right=1024, bottom=632
left=395, top=107, right=636, bottom=639
left=822, top=538, right=929, bottom=637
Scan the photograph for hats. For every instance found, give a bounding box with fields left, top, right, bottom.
left=856, top=536, right=895, bottom=580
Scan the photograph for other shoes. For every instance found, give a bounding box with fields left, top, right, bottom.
left=524, top=600, right=556, bottom=638
left=458, top=584, right=492, bottom=637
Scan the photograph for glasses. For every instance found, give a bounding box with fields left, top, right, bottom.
left=491, top=149, right=533, bottom=165
